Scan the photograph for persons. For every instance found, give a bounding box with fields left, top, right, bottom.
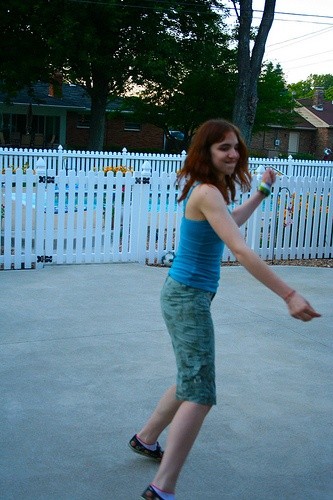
left=127, top=119, right=323, bottom=500
left=322, top=144, right=333, bottom=161
left=1, top=121, right=12, bottom=144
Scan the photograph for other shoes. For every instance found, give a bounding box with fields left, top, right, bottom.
left=128, top=432, right=165, bottom=463
left=140, top=484, right=165, bottom=500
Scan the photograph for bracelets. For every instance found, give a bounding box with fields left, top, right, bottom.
left=284, top=289, right=296, bottom=300
left=257, top=180, right=271, bottom=196
